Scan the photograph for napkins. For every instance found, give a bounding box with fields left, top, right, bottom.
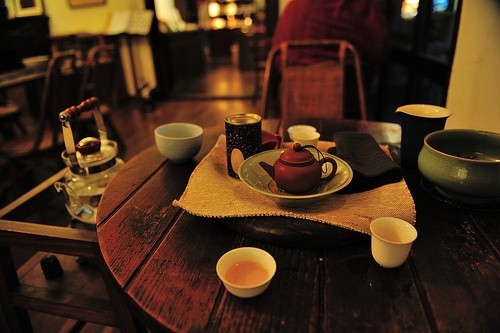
left=327, top=131, right=403, bottom=195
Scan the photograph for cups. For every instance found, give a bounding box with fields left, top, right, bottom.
left=370, top=217, right=418, bottom=267
left=287, top=126, right=320, bottom=147
left=395, top=103, right=452, bottom=179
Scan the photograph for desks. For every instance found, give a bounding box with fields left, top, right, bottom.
left=97, top=117, right=500, bottom=333
left=0, top=57, right=111, bottom=93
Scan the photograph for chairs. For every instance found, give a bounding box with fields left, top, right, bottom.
left=262, top=39, right=366, bottom=121
left=0, top=35, right=122, bottom=196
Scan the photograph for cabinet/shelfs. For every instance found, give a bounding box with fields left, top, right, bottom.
left=0, top=166, right=149, bottom=333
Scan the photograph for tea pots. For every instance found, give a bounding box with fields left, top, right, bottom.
left=55, top=97, right=125, bottom=224
left=258, top=143, right=338, bottom=195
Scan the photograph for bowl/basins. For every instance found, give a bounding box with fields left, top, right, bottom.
left=216, top=247, right=276, bottom=298
left=418, top=129, right=500, bottom=203
left=238, top=149, right=353, bottom=207
left=155, top=123, right=203, bottom=163
